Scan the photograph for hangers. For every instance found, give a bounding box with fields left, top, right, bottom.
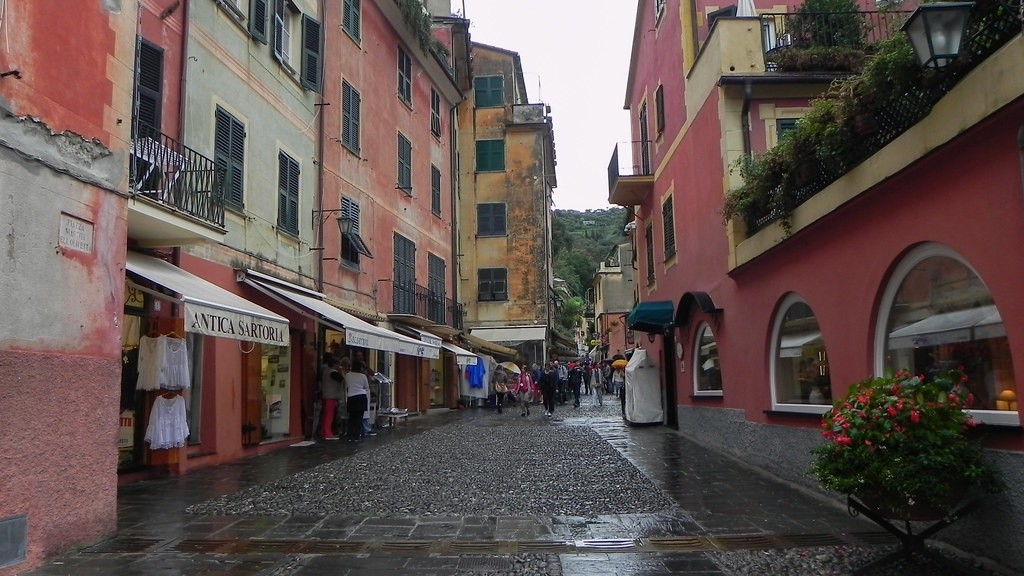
left=165, top=327, right=184, bottom=340
left=144, top=322, right=166, bottom=337
left=158, top=389, right=178, bottom=399
left=370, top=372, right=393, bottom=385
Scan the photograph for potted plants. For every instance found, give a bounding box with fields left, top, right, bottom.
left=717, top=33, right=914, bottom=234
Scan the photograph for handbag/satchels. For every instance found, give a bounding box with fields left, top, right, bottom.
left=495, top=382, right=508, bottom=393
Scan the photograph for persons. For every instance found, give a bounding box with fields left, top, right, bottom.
left=700, top=366, right=722, bottom=389
left=801, top=355, right=829, bottom=403
left=491, top=366, right=507, bottom=413
left=317, top=353, right=377, bottom=441
left=926, top=350, right=993, bottom=406
left=508, top=359, right=625, bottom=416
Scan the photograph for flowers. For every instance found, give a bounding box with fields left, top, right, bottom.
left=803, top=369, right=1010, bottom=521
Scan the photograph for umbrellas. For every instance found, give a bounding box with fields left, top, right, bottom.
left=611, top=360, right=628, bottom=368
left=603, top=359, right=613, bottom=362
left=500, top=361, right=521, bottom=375
left=701, top=357, right=717, bottom=370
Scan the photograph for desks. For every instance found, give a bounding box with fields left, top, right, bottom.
left=377, top=413, right=409, bottom=429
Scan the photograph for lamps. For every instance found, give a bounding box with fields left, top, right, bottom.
left=310, top=207, right=354, bottom=239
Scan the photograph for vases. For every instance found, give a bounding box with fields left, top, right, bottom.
left=873, top=487, right=947, bottom=523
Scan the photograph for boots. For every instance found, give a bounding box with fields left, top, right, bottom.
left=497, top=402, right=502, bottom=413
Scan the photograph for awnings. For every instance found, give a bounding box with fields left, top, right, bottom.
left=246, top=277, right=440, bottom=360
left=471, top=327, right=546, bottom=341
left=589, top=345, right=598, bottom=358
left=628, top=301, right=674, bottom=334
left=397, top=323, right=443, bottom=348
left=553, top=329, right=580, bottom=360
left=125, top=251, right=289, bottom=347
left=443, top=342, right=478, bottom=365
left=886, top=301, right=1007, bottom=348
left=777, top=328, right=822, bottom=358
left=700, top=342, right=717, bottom=355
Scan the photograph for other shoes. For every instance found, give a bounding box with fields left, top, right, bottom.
left=322, top=436, right=325, bottom=439
left=354, top=439, right=359, bottom=442
left=348, top=439, right=352, bottom=441
left=527, top=411, right=530, bottom=415
left=545, top=410, right=548, bottom=415
left=325, top=436, right=340, bottom=440
left=548, top=412, right=552, bottom=416
left=521, top=413, right=525, bottom=417
